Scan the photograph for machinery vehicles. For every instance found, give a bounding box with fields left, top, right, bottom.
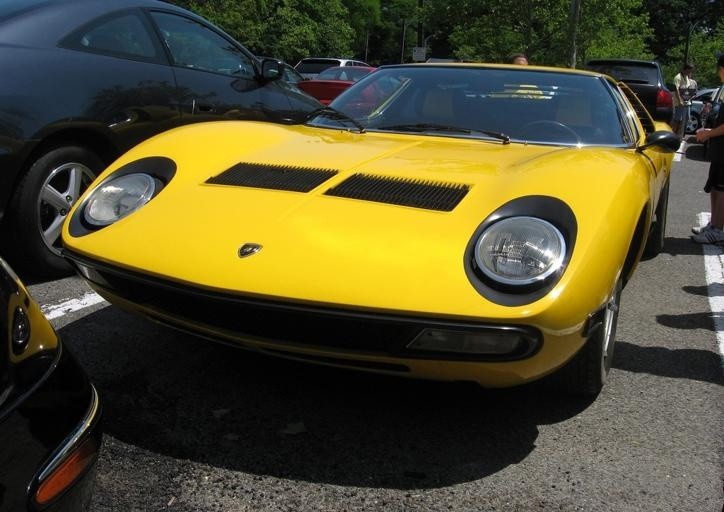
left=60, top=61, right=679, bottom=398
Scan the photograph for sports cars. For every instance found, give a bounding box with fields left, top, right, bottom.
left=60, top=61, right=679, bottom=398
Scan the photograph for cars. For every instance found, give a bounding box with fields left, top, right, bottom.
left=584, top=59, right=673, bottom=133
left=677, top=89, right=723, bottom=134
left=1, top=1, right=330, bottom=271
left=257, top=55, right=402, bottom=122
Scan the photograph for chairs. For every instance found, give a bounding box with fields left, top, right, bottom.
left=692, top=222, right=724, bottom=246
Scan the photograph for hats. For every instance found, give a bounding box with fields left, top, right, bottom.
left=584, top=59, right=673, bottom=133
left=677, top=89, right=723, bottom=134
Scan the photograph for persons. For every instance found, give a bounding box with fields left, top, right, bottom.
left=691, top=56, right=724, bottom=244
left=671, top=64, right=692, bottom=142
left=688, top=72, right=697, bottom=89
left=490, top=52, right=544, bottom=98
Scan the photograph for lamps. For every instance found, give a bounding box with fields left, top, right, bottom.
left=412, top=88, right=466, bottom=129
left=538, top=96, right=607, bottom=144
